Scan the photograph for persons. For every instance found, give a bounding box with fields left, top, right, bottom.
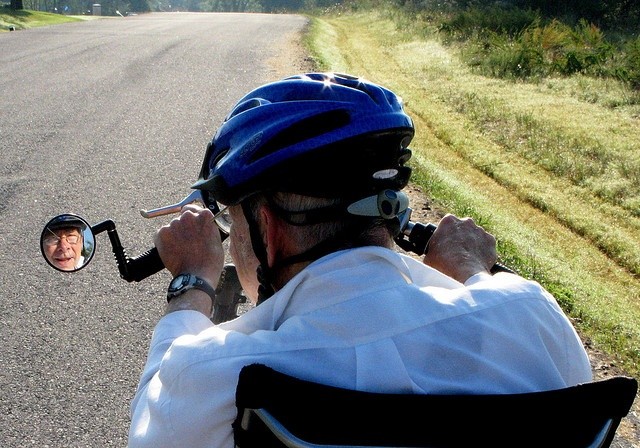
left=127, top=72, right=596, bottom=447
left=42, top=215, right=87, bottom=269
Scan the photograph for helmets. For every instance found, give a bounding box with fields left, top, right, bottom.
left=43, top=214, right=88, bottom=263
left=189, top=70, right=416, bottom=309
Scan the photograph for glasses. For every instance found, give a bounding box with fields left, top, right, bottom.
left=206, top=201, right=232, bottom=233
left=43, top=233, right=83, bottom=244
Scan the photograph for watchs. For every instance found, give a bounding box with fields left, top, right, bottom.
left=167, top=273, right=215, bottom=301
